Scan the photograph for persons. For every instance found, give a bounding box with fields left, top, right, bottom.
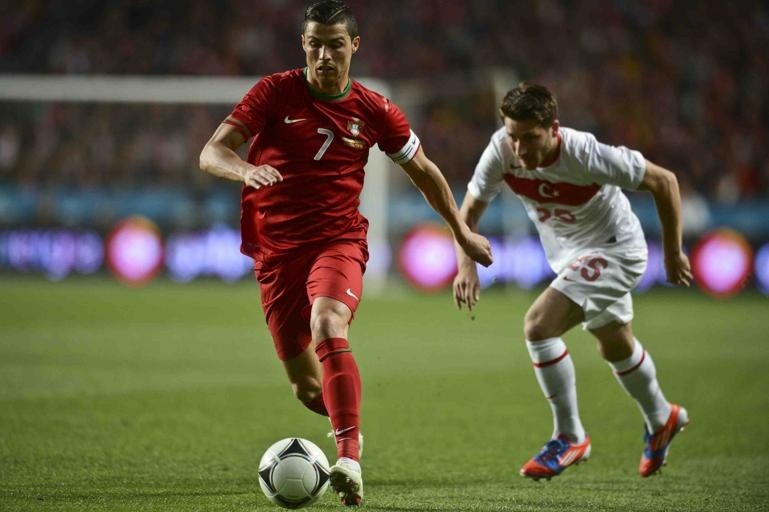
left=200, top=1, right=494, bottom=503
left=2, top=0, right=768, bottom=204
left=451, top=83, right=694, bottom=481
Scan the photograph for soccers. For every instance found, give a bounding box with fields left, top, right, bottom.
left=257, top=438, right=331, bottom=509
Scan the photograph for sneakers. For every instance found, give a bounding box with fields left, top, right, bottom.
left=328, top=456, right=364, bottom=504
left=521, top=432, right=592, bottom=481
left=327, top=429, right=363, bottom=461
left=638, top=403, right=689, bottom=477
